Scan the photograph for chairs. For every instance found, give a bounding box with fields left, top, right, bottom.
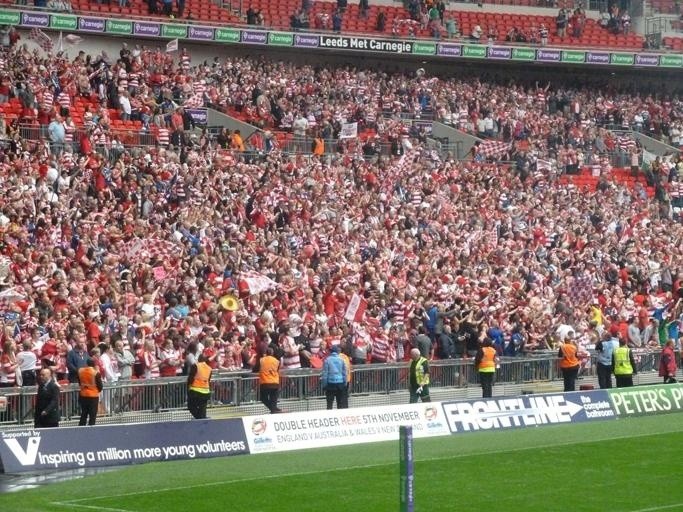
left=556, top=167, right=657, bottom=198
left=70, top=1, right=645, bottom=49
left=661, top=35, right=682, bottom=51
left=2, top=85, right=144, bottom=134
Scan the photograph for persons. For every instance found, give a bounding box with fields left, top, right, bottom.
left=34, top=368, right=59, bottom=428
left=77, top=359, right=102, bottom=426
